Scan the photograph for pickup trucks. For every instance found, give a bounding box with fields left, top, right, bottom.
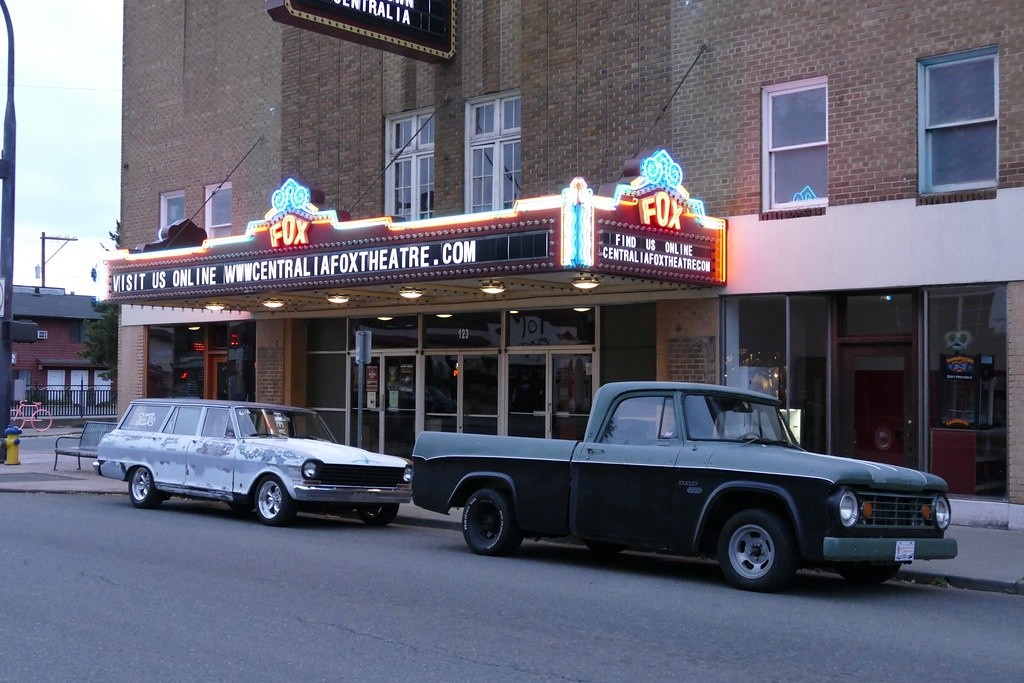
left=410, top=379, right=958, bottom=594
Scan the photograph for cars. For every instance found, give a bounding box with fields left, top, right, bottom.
left=92, top=398, right=414, bottom=528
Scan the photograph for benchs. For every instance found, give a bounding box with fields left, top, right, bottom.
left=53, top=421, right=118, bottom=471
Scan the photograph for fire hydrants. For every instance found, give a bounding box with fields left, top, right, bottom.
left=4, top=425, right=23, bottom=465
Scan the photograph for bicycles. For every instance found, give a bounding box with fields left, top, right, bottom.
left=10, top=399, right=53, bottom=432
